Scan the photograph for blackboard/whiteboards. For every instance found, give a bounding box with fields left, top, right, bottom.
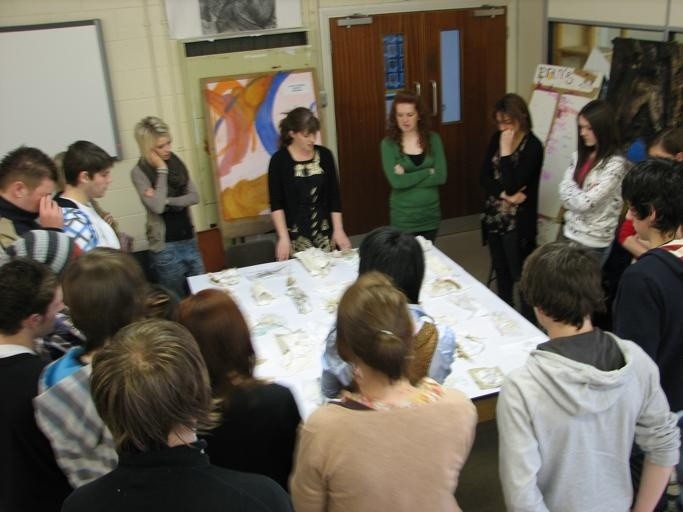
left=0, top=18, right=123, bottom=168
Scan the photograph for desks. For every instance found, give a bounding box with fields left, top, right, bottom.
left=187, top=234, right=551, bottom=424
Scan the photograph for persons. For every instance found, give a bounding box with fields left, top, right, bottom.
left=130, top=117, right=206, bottom=300
left=381, top=91, right=447, bottom=243
left=267, top=107, right=351, bottom=259
left=1, top=141, right=302, bottom=512
left=481, top=93, right=544, bottom=320
left=321, top=226, right=456, bottom=399
left=495, top=238, right=680, bottom=511
left=288, top=274, right=479, bottom=512
left=556, top=97, right=683, bottom=433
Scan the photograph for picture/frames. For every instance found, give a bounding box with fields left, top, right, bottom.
left=198, top=67, right=328, bottom=240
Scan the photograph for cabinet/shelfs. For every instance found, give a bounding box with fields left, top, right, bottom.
left=540, top=18, right=683, bottom=81
